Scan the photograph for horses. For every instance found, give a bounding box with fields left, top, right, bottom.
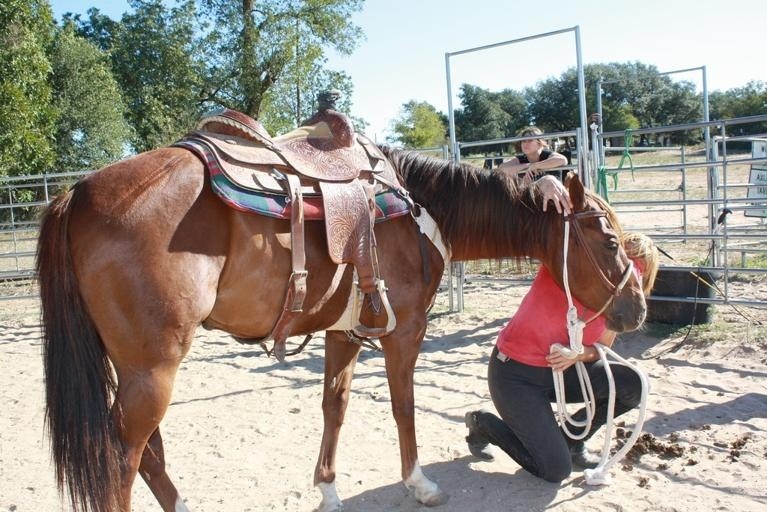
left=29, top=109, right=650, bottom=512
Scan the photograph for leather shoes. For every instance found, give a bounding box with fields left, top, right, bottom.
left=462, top=409, right=493, bottom=462
left=568, top=441, right=600, bottom=471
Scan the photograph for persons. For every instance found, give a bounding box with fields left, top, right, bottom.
left=464, top=174, right=661, bottom=486
left=497, top=126, right=571, bottom=183
